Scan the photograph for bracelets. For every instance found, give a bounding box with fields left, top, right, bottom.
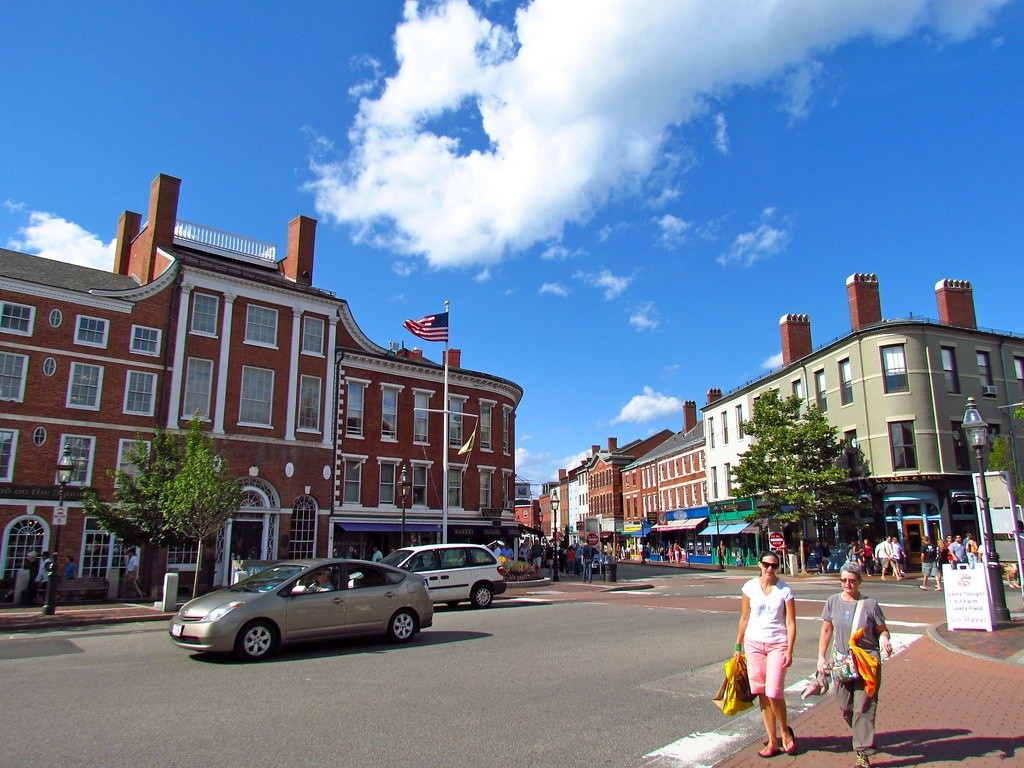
left=734, top=642, right=742, bottom=652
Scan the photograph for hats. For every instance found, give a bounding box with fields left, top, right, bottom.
left=815, top=538, right=822, bottom=544
left=821, top=539, right=826, bottom=542
left=505, top=543, right=510, bottom=546
left=126, top=547, right=136, bottom=552
left=41, top=552, right=50, bottom=556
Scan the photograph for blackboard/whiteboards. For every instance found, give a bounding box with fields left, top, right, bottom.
left=942, top=563, right=995, bottom=632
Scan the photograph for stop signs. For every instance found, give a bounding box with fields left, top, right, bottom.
left=769, top=533, right=783, bottom=547
left=586, top=534, right=598, bottom=545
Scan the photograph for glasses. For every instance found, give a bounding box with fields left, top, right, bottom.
left=840, top=578, right=856, bottom=583
left=317, top=572, right=325, bottom=576
left=761, top=562, right=779, bottom=569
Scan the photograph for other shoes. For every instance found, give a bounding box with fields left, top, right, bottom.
left=897, top=577, right=902, bottom=582
left=919, top=585, right=926, bottom=590
left=934, top=585, right=941, bottom=591
left=880, top=577, right=887, bottom=580
left=855, top=753, right=871, bottom=768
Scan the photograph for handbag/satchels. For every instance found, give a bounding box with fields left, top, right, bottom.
left=971, top=540, right=978, bottom=555
left=711, top=654, right=758, bottom=715
left=832, top=649, right=862, bottom=682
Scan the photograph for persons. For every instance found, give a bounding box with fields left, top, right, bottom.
left=1006, top=562, right=1021, bottom=588
left=816, top=560, right=893, bottom=768
left=308, top=568, right=335, bottom=593
left=716, top=540, right=726, bottom=568
left=733, top=550, right=797, bottom=758
left=371, top=545, right=383, bottom=563
left=25, top=551, right=77, bottom=607
left=118, top=548, right=143, bottom=598
left=1017, top=519, right=1024, bottom=564
left=801, top=532, right=978, bottom=592
left=493, top=537, right=680, bottom=583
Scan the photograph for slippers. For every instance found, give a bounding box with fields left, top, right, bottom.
left=783, top=726, right=795, bottom=751
left=758, top=744, right=782, bottom=757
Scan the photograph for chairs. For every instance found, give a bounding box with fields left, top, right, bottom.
left=414, top=556, right=424, bottom=571
left=428, top=555, right=439, bottom=570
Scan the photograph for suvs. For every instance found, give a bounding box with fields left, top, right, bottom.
left=377, top=544, right=507, bottom=609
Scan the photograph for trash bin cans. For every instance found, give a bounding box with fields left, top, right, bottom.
left=603, top=560, right=617, bottom=582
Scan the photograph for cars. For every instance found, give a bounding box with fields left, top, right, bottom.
left=563, top=546, right=606, bottom=573
left=168, top=558, right=434, bottom=660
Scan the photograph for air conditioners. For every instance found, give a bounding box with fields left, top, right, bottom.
left=982, top=386, right=997, bottom=395
left=504, top=501, right=514, bottom=509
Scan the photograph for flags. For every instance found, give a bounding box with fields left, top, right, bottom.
left=458, top=419, right=479, bottom=455
left=403, top=312, right=449, bottom=343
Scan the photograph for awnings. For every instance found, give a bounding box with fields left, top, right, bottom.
left=518, top=523, right=544, bottom=538
left=621, top=528, right=652, bottom=537
left=337, top=524, right=443, bottom=532
left=651, top=517, right=708, bottom=532
left=698, top=522, right=751, bottom=535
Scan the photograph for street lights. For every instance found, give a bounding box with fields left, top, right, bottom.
left=397, top=465, right=411, bottom=549
left=640, top=518, right=646, bottom=563
left=960, top=397, right=1012, bottom=620
left=714, top=501, right=724, bottom=568
left=549, top=489, right=561, bottom=581
left=42, top=442, right=76, bottom=614
